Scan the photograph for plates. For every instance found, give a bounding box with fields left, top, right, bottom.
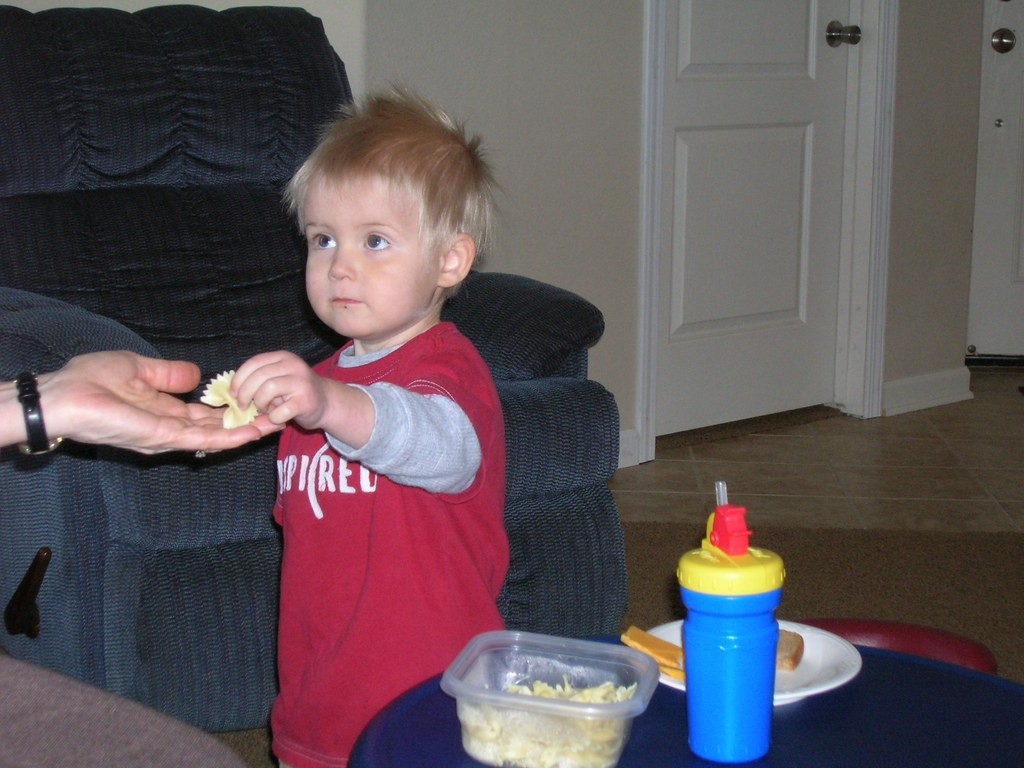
left=646, top=619, right=863, bottom=707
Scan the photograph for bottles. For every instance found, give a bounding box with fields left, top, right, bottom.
left=676, top=482, right=787, bottom=765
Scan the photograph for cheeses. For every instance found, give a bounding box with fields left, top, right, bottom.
left=619, top=625, right=685, bottom=682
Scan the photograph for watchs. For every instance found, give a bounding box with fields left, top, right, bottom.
left=14, top=372, right=61, bottom=454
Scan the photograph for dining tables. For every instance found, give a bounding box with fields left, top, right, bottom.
left=348, top=635, right=1024, bottom=768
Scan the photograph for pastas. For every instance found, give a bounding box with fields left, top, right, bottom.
left=200, top=369, right=260, bottom=429
left=462, top=675, right=635, bottom=768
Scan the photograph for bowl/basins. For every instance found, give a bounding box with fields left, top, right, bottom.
left=439, top=629, right=661, bottom=768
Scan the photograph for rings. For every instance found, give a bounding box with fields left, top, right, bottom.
left=195, top=450, right=205, bottom=458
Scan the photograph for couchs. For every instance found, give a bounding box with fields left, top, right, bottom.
left=0, top=4, right=624, bottom=733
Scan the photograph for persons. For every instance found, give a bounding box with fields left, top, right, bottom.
left=231, top=93, right=510, bottom=768
left=0, top=348, right=286, bottom=767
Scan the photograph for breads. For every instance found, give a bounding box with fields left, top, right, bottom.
left=774, top=629, right=805, bottom=669
